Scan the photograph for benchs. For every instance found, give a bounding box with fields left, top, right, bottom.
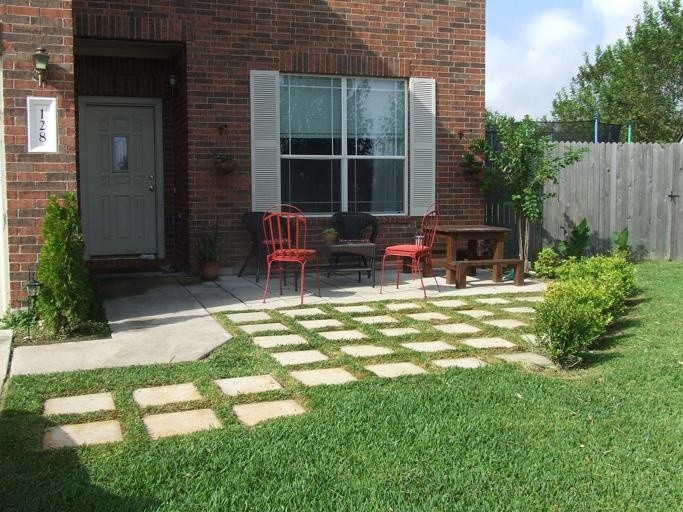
left=443, top=258, right=525, bottom=290
left=402, top=246, right=470, bottom=273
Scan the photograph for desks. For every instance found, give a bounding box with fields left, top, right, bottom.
left=421, top=223, right=513, bottom=284
left=284, top=242, right=376, bottom=288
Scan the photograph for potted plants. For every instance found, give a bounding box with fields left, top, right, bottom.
left=191, top=210, right=227, bottom=281
left=321, top=223, right=338, bottom=245
left=214, top=150, right=238, bottom=174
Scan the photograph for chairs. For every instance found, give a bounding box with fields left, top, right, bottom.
left=262, top=203, right=321, bottom=307
left=327, top=211, right=379, bottom=282
left=379, top=200, right=443, bottom=298
left=238, top=211, right=298, bottom=291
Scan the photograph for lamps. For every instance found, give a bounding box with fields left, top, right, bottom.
left=32, top=43, right=49, bottom=87
left=168, top=72, right=177, bottom=96
